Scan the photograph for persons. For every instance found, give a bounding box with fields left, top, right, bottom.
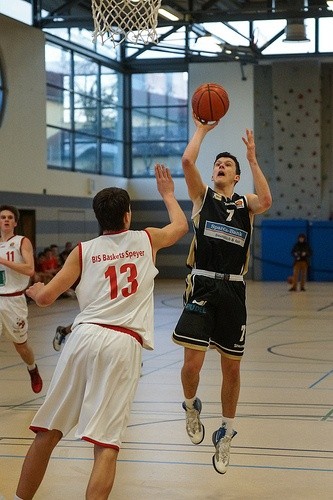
left=1, top=204, right=43, bottom=394
left=28, top=242, right=76, bottom=297
left=288, top=233, right=313, bottom=291
left=11, top=163, right=188, bottom=500
left=171, top=109, right=273, bottom=475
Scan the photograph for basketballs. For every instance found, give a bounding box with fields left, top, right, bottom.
left=190, top=82, right=231, bottom=124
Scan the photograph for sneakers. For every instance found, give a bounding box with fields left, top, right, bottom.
left=182, top=397, right=205, bottom=445
left=212, top=426, right=237, bottom=474
left=53, top=326, right=66, bottom=351
left=27, top=364, right=42, bottom=393
